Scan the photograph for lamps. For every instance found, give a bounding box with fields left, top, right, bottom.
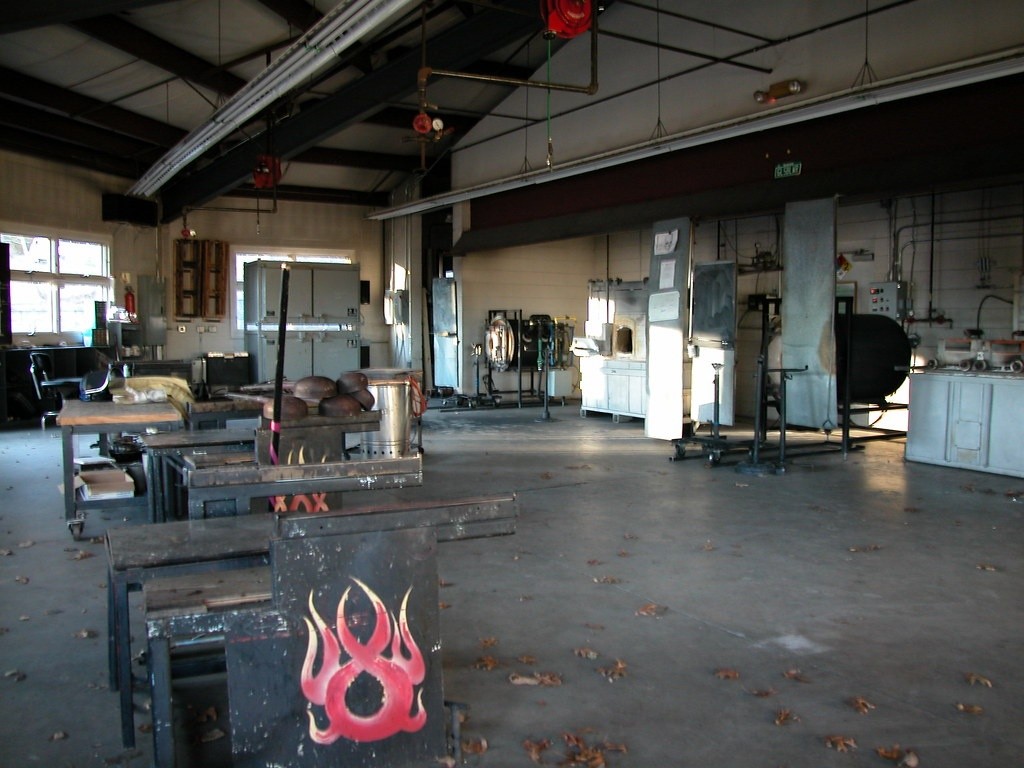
left=753, top=79, right=802, bottom=106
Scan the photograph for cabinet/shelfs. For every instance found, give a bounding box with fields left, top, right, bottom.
left=904, top=373, right=1023, bottom=477
left=1, top=346, right=112, bottom=431
left=107, top=322, right=143, bottom=362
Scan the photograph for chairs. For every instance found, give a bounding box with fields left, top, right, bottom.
left=30, top=352, right=82, bottom=430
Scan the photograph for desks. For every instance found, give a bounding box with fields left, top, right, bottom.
left=57, top=393, right=277, bottom=768
